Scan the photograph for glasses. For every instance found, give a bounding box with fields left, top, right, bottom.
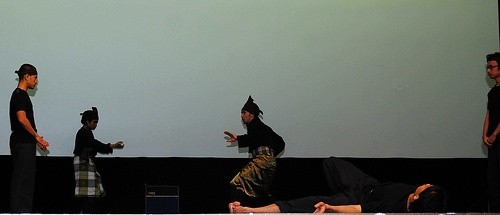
left=487, top=65, right=498, bottom=69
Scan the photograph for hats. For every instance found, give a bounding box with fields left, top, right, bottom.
left=80, top=107, right=98, bottom=120
left=15, top=64, right=38, bottom=75
left=241, top=95, right=263, bottom=115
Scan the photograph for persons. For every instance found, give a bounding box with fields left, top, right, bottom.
left=482, top=52, right=500, bottom=215
left=73, top=107, right=124, bottom=214
left=9, top=64, right=49, bottom=214
left=229, top=156, right=449, bottom=213
left=224, top=95, right=286, bottom=208
